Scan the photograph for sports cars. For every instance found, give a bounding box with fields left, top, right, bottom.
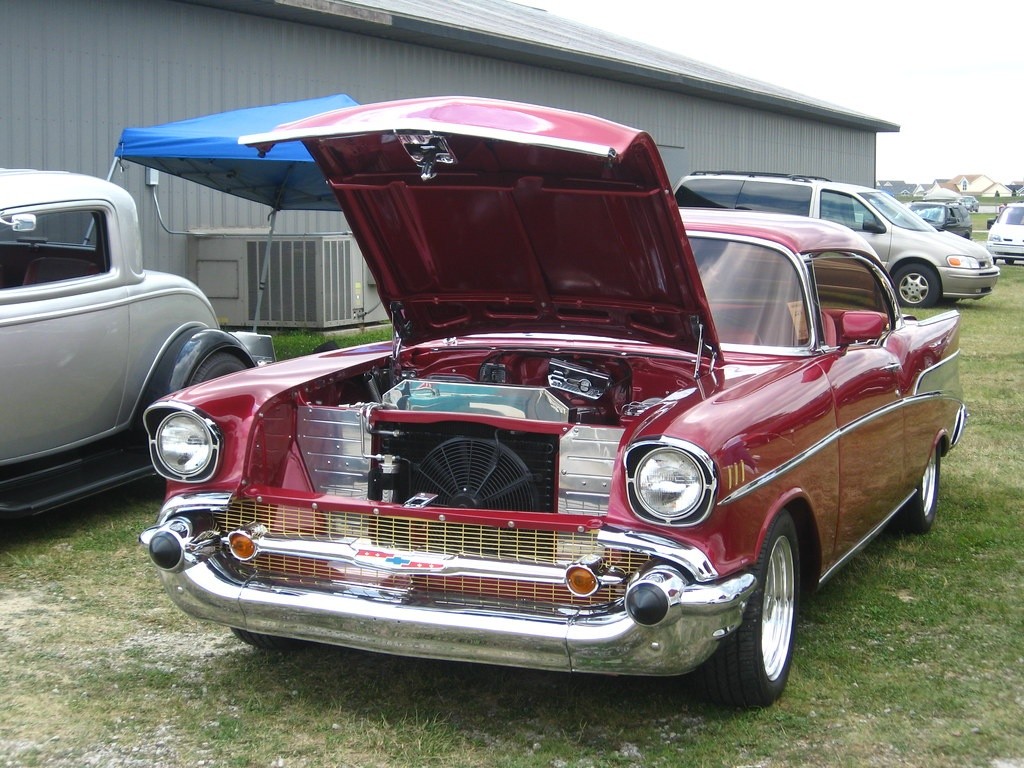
left=142, top=96, right=961, bottom=708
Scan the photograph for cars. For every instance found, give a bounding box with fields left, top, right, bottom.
left=0, top=167, right=277, bottom=518
left=986, top=202, right=1024, bottom=265
left=957, top=196, right=979, bottom=212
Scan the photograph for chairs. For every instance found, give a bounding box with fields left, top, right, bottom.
left=826, top=200, right=847, bottom=226
left=755, top=308, right=836, bottom=349
left=21, top=256, right=101, bottom=286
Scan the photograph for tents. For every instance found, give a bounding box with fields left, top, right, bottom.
left=114, top=92, right=361, bottom=211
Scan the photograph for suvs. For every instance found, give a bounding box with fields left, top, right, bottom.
left=894, top=200, right=971, bottom=239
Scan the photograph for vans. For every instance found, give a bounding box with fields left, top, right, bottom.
left=671, top=171, right=997, bottom=308
